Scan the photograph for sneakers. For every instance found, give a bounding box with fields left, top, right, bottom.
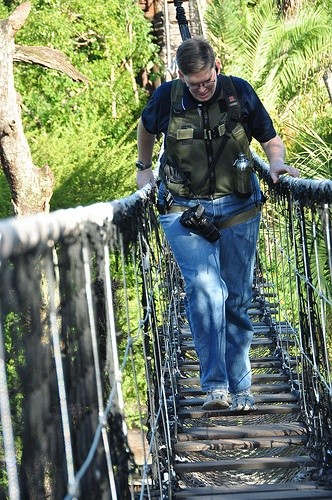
left=201, top=389, right=258, bottom=412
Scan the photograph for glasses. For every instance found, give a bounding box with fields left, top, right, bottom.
left=184, top=70, right=216, bottom=91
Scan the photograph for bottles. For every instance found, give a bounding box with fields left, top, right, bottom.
left=234, top=154, right=251, bottom=193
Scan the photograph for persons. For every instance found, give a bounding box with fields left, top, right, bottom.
left=136, top=38, right=299, bottom=411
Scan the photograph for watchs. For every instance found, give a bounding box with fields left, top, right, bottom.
left=136, top=161, right=152, bottom=171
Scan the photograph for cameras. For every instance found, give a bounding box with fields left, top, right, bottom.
left=179, top=204, right=221, bottom=243
